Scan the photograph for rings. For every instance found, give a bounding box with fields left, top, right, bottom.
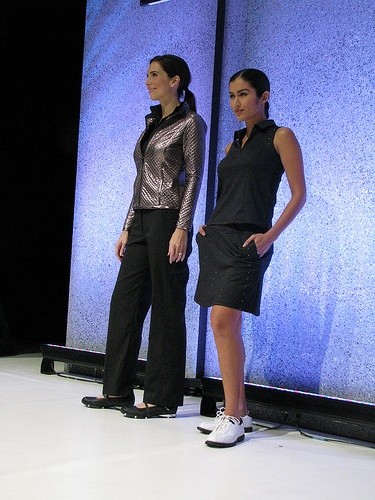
left=178, top=253, right=183, bottom=256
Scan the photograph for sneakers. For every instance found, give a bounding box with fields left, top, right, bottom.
left=205, top=415, right=245, bottom=446
left=197, top=406, right=252, bottom=433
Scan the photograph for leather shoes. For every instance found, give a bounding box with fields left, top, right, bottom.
left=81, top=395, right=135, bottom=408
left=121, top=402, right=177, bottom=417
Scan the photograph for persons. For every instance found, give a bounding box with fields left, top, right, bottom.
left=80, top=53, right=208, bottom=417
left=195, top=68, right=306, bottom=448
